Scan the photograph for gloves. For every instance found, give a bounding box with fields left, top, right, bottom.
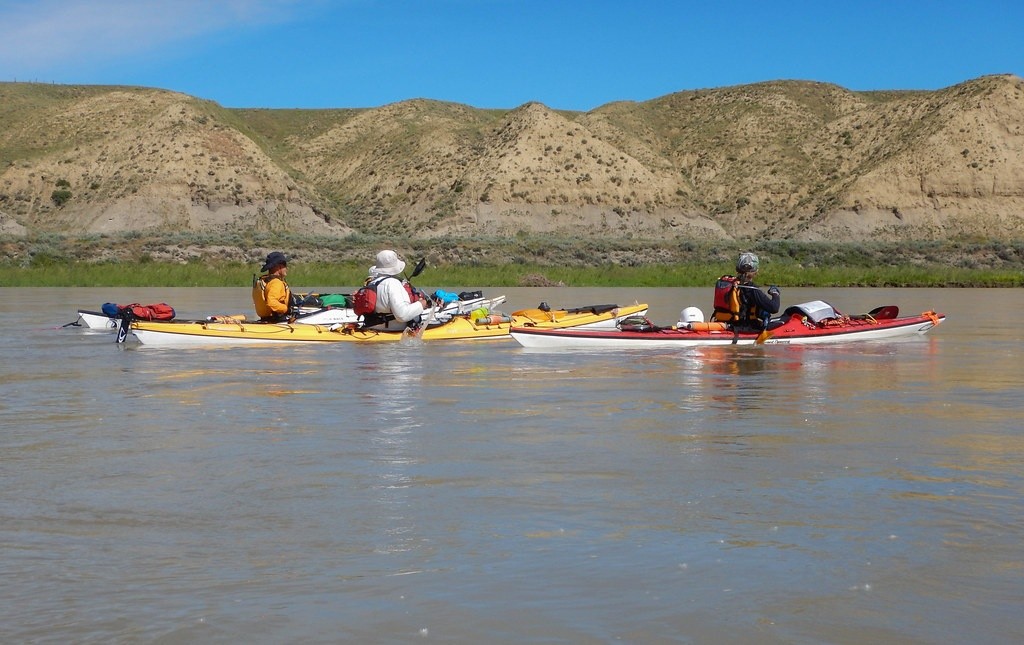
left=767, top=286, right=780, bottom=295
left=290, top=305, right=299, bottom=314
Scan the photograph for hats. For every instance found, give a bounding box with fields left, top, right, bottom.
left=261, top=251, right=294, bottom=272
left=736, top=253, right=759, bottom=273
left=369, top=250, right=405, bottom=275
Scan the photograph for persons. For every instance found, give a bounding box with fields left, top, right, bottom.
left=261, top=251, right=303, bottom=323
left=363, top=249, right=426, bottom=329
left=726, top=251, right=780, bottom=333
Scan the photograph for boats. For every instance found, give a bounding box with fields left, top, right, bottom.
left=74, top=292, right=508, bottom=330
left=133, top=299, right=653, bottom=348
left=507, top=308, right=949, bottom=349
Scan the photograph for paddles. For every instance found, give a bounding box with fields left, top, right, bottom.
left=754, top=317, right=772, bottom=346
left=401, top=320, right=428, bottom=347
left=285, top=291, right=313, bottom=323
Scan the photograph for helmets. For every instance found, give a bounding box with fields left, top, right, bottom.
left=680, top=306, right=704, bottom=323
